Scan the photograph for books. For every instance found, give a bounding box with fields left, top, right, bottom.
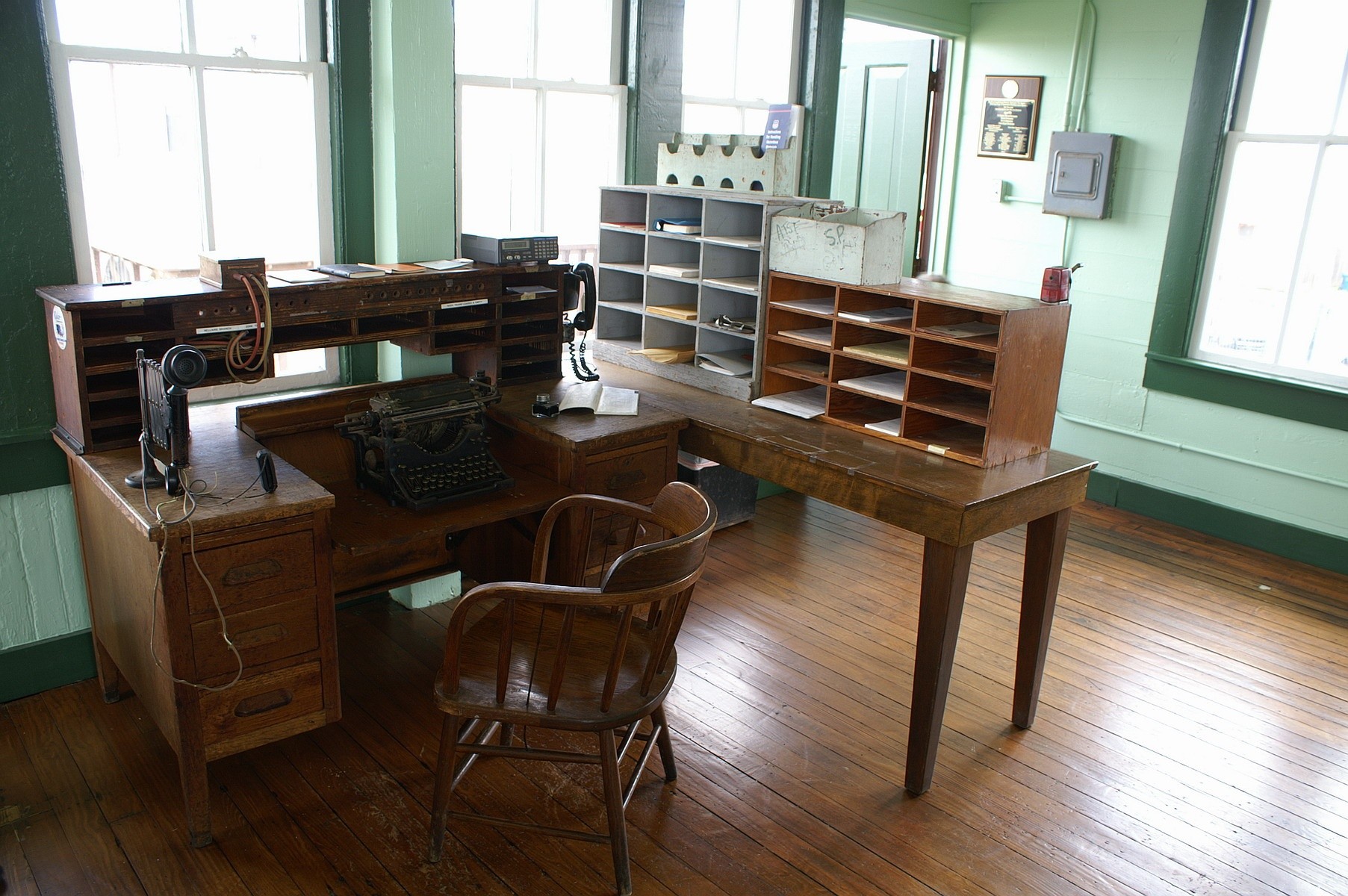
left=413, top=259, right=471, bottom=270
left=317, top=264, right=385, bottom=279
left=358, top=263, right=426, bottom=275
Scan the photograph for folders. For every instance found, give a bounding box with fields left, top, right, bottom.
left=694, top=349, right=753, bottom=375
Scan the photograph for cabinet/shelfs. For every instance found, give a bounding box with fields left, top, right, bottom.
left=31, top=257, right=576, bottom=457
left=761, top=262, right=1075, bottom=471
left=591, top=182, right=846, bottom=404
left=769, top=203, right=908, bottom=286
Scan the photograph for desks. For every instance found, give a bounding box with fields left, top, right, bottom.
left=49, top=376, right=691, bottom=852
left=561, top=346, right=1099, bottom=802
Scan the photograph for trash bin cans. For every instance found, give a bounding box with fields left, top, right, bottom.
left=677, top=450, right=759, bottom=531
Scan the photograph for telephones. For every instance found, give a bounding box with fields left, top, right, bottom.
left=573, top=264, right=597, bottom=332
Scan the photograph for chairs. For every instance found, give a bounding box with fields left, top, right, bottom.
left=424, top=475, right=722, bottom=896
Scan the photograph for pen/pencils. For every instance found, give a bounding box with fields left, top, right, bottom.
left=102, top=282, right=132, bottom=286
